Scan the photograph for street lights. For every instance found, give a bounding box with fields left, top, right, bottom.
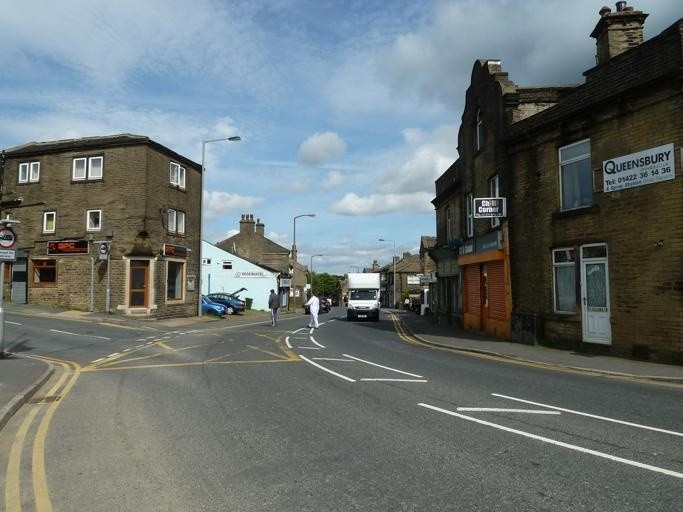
left=350, top=265, right=359, bottom=273
left=310, top=254, right=323, bottom=303
left=293, top=212, right=317, bottom=314
left=378, top=238, right=396, bottom=308
left=198, top=136, right=242, bottom=318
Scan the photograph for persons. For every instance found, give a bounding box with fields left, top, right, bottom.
left=304, top=292, right=319, bottom=329
left=269, top=289, right=280, bottom=327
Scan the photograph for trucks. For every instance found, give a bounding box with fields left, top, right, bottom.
left=346, top=272, right=381, bottom=322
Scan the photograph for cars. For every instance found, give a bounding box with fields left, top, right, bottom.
left=205, top=286, right=248, bottom=315
left=303, top=297, right=333, bottom=314
left=200, top=293, right=228, bottom=317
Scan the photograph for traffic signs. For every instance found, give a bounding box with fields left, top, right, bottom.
left=0, top=250, right=17, bottom=263
left=99, top=254, right=108, bottom=259
